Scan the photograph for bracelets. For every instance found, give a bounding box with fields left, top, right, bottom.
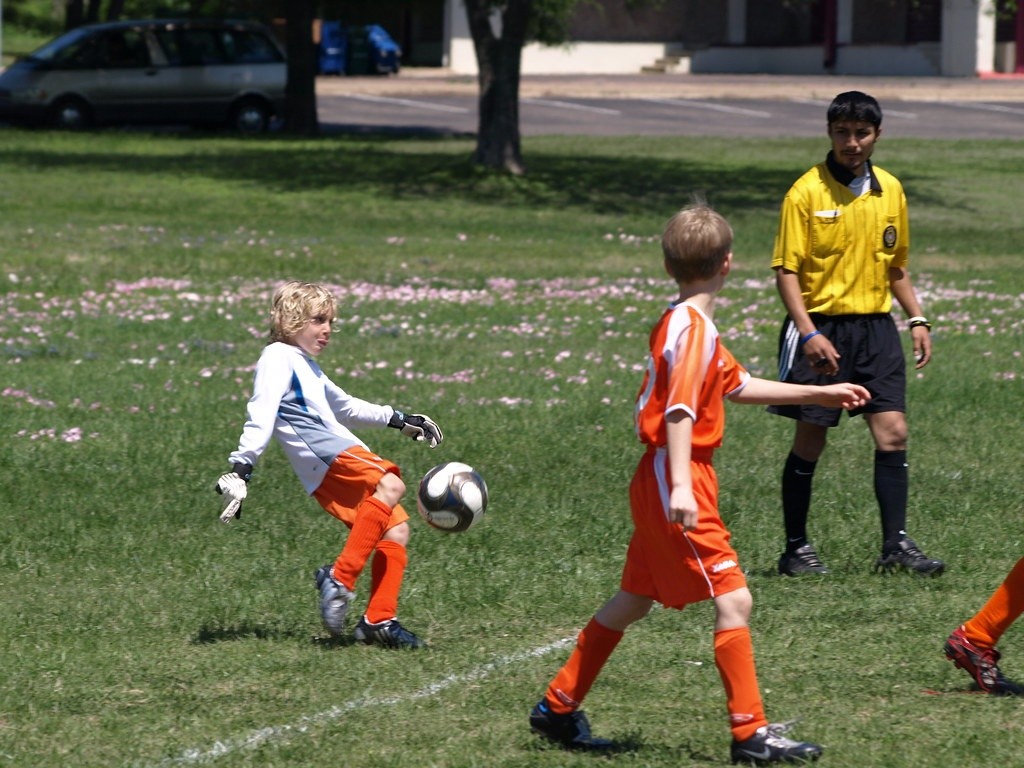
left=800, top=331, right=823, bottom=345
left=907, top=316, right=933, bottom=331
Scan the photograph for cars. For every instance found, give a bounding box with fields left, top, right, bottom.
left=315, top=19, right=405, bottom=79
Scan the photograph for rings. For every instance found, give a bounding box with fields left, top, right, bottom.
left=816, top=358, right=829, bottom=368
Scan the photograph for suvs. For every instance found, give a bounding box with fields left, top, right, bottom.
left=0, top=14, right=292, bottom=142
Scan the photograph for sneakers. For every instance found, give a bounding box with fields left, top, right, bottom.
left=529, top=697, right=620, bottom=752
left=876, top=530, right=946, bottom=578
left=944, top=623, right=1011, bottom=695
left=315, top=562, right=356, bottom=636
left=730, top=722, right=824, bottom=766
left=777, top=542, right=834, bottom=581
left=350, top=614, right=430, bottom=652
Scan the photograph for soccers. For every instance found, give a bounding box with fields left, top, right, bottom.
left=418, top=462, right=488, bottom=533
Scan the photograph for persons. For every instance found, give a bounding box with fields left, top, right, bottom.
left=772, top=91, right=946, bottom=580
left=946, top=559, right=1024, bottom=690
left=526, top=208, right=875, bottom=765
left=216, top=282, right=443, bottom=655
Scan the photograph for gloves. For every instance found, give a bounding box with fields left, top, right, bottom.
left=215, top=460, right=254, bottom=522
left=387, top=408, right=444, bottom=449
